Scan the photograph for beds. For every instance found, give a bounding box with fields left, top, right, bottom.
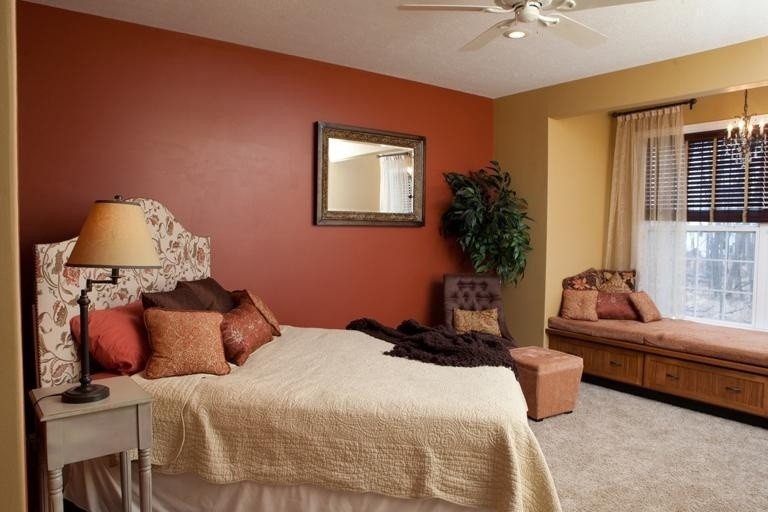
left=30, top=198, right=562, bottom=512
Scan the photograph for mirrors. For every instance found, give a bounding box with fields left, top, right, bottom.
left=310, top=119, right=429, bottom=229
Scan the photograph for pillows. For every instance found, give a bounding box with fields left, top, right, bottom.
left=177, top=274, right=238, bottom=312
left=247, top=289, right=280, bottom=331
left=599, top=269, right=637, bottom=293
left=220, top=300, right=274, bottom=368
left=596, top=269, right=634, bottom=294
left=628, top=290, right=664, bottom=324
left=233, top=288, right=282, bottom=337
left=560, top=289, right=600, bottom=322
left=69, top=297, right=151, bottom=377
left=140, top=279, right=206, bottom=311
left=562, top=267, right=602, bottom=292
left=596, top=292, right=639, bottom=320
left=452, top=307, right=504, bottom=339
left=142, top=306, right=232, bottom=380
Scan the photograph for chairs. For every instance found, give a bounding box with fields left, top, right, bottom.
left=442, top=272, right=519, bottom=350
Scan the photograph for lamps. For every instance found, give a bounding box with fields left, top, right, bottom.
left=59, top=194, right=164, bottom=406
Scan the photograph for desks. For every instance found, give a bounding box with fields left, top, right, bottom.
left=29, top=372, right=164, bottom=512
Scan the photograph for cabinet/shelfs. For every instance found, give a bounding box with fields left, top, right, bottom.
left=543, top=326, right=766, bottom=431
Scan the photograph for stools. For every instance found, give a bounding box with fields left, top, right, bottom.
left=508, top=344, right=586, bottom=422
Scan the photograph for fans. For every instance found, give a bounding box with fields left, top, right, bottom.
left=392, top=0, right=648, bottom=52
